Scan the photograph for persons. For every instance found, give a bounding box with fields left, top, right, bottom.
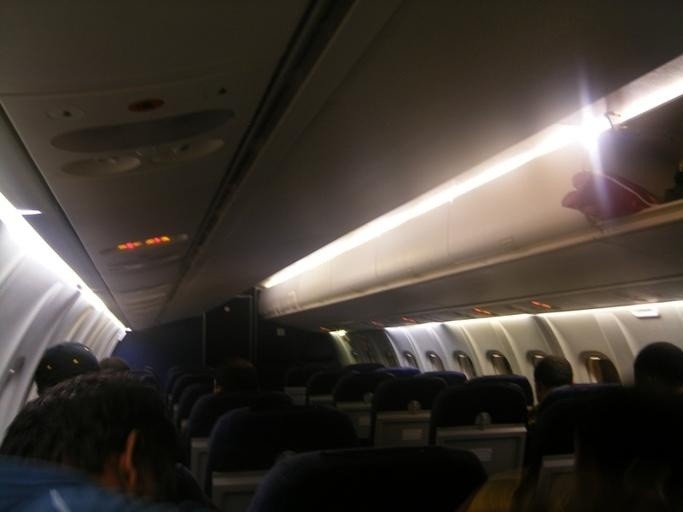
left=635, top=342, right=683, bottom=385
left=527, top=356, right=573, bottom=429
left=287, top=405, right=360, bottom=457
left=0, top=367, right=175, bottom=512
left=99, top=358, right=133, bottom=369
left=214, top=357, right=259, bottom=395
left=34, top=342, right=100, bottom=397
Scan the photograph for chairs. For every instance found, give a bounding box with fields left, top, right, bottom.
left=1, top=361, right=683, bottom=507
